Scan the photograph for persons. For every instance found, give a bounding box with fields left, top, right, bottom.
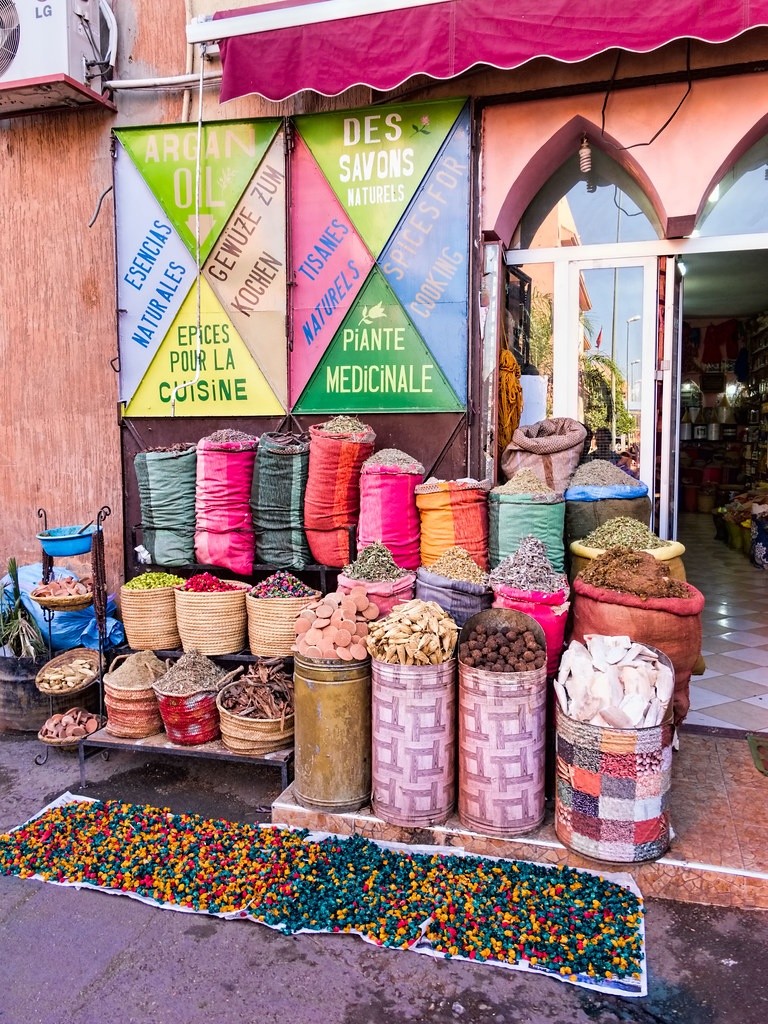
left=581, top=426, right=640, bottom=479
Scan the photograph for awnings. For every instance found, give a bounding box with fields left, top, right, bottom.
left=185, top=0, right=768, bottom=104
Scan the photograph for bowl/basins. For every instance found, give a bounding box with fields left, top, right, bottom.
left=36, top=525, right=104, bottom=557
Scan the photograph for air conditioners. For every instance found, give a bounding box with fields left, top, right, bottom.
left=0, top=0, right=102, bottom=114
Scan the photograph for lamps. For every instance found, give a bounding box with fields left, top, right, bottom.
left=580, top=131, right=591, bottom=171
left=677, top=254, right=687, bottom=276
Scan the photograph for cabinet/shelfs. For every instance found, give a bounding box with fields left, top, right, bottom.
left=743, top=320, right=768, bottom=491
left=34, top=505, right=111, bottom=765
left=72, top=526, right=351, bottom=794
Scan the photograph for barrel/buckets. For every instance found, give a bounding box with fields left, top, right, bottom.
left=293, top=650, right=371, bottom=814
left=370, top=659, right=457, bottom=828
left=457, top=608, right=548, bottom=839
left=707, top=424, right=720, bottom=441
left=554, top=641, right=675, bottom=866
left=702, top=464, right=721, bottom=484
left=693, top=426, right=707, bottom=439
left=680, top=423, right=692, bottom=440
